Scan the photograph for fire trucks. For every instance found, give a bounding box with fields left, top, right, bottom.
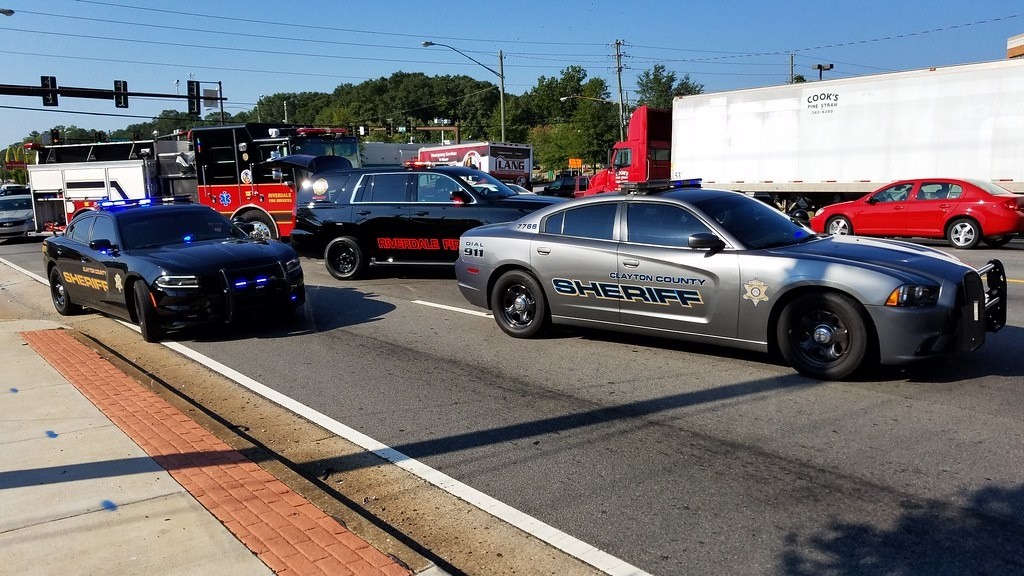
left=24, top=122, right=363, bottom=247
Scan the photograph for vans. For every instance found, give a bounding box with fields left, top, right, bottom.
left=561, top=175, right=595, bottom=200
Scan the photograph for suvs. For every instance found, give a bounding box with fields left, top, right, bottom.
left=256, top=154, right=583, bottom=280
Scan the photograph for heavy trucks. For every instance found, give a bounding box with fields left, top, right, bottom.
left=583, top=57, right=1024, bottom=227
left=418, top=142, right=533, bottom=191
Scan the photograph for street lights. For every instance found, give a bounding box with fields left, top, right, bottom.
left=421, top=40, right=506, bottom=143
left=559, top=95, right=625, bottom=164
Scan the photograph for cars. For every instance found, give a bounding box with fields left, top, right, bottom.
left=0, top=195, right=33, bottom=239
left=0, top=184, right=31, bottom=196
left=809, top=178, right=1024, bottom=251
left=453, top=179, right=1007, bottom=384
left=544, top=177, right=577, bottom=198
left=42, top=201, right=306, bottom=344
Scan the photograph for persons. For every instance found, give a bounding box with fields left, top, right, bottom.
left=94, top=133, right=102, bottom=144
left=466, top=157, right=478, bottom=170
left=179, top=127, right=189, bottom=140
